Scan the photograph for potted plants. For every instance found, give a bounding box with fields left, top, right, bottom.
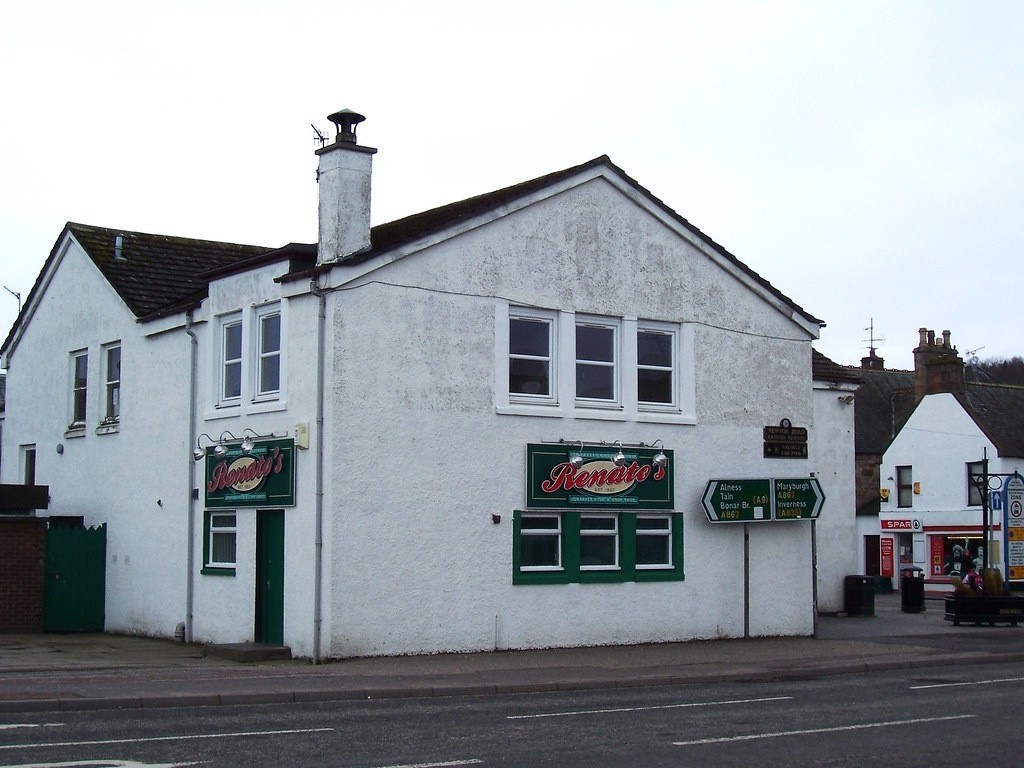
left=944, top=568, right=1024, bottom=627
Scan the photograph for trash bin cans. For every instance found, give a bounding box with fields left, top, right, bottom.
left=845, top=575, right=874, bottom=617
left=900, top=566, right=926, bottom=612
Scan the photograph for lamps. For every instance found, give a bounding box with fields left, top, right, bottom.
left=839, top=394, right=856, bottom=403
left=641, top=439, right=667, bottom=467
left=560, top=439, right=585, bottom=469
left=241, top=428, right=272, bottom=457
left=215, top=431, right=249, bottom=459
left=193, top=433, right=226, bottom=461
left=888, top=476, right=893, bottom=480
left=602, top=440, right=625, bottom=467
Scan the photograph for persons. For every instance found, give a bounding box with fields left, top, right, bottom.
left=960, top=549, right=984, bottom=589
